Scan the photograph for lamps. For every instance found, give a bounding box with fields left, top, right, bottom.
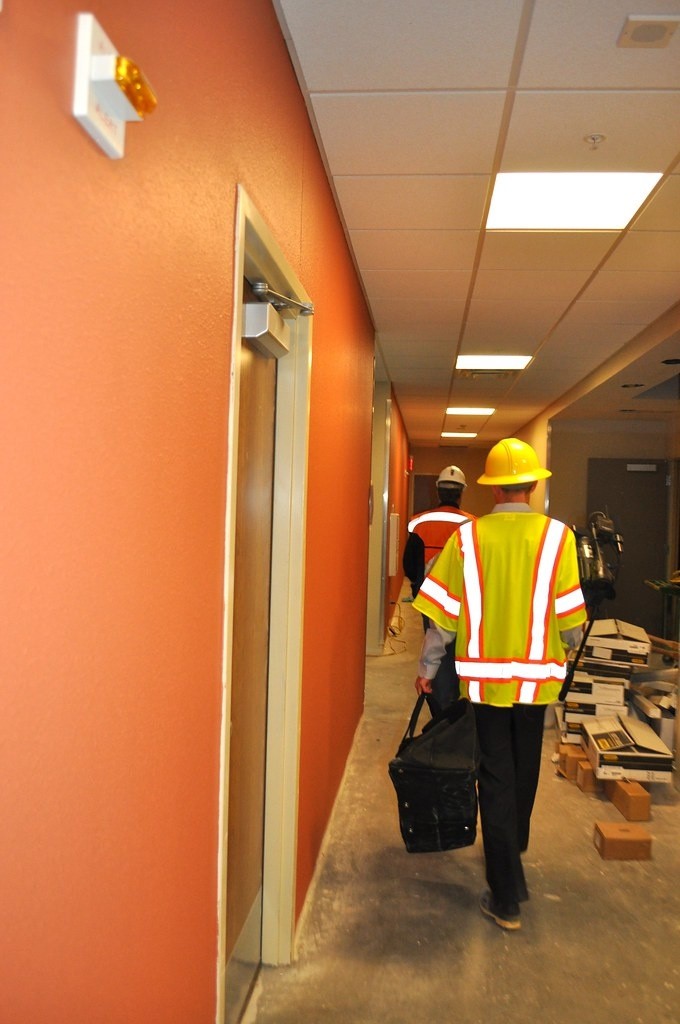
left=74, top=10, right=159, bottom=160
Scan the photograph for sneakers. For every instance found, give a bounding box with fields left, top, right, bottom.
left=480, top=890, right=521, bottom=929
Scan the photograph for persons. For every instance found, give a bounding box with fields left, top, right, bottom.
left=411, top=438, right=587, bottom=928
left=402, top=466, right=479, bottom=719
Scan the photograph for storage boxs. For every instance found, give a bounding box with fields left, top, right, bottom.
left=390, top=700, right=476, bottom=853
left=555, top=619, right=680, bottom=861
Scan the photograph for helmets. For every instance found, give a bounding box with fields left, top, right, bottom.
left=477, top=438, right=552, bottom=485
left=436, top=465, right=467, bottom=491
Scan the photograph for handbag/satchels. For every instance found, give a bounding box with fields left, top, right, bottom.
left=389, top=694, right=480, bottom=853
